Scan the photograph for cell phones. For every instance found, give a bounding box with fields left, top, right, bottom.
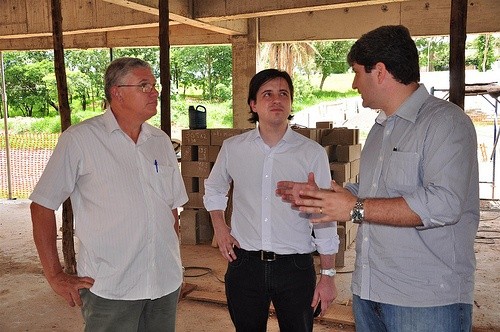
left=314, top=300, right=322, bottom=318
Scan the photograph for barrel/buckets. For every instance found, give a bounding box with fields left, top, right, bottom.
left=189, top=105, right=206, bottom=129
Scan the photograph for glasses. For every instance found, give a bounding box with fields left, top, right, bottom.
left=112, top=80, right=159, bottom=94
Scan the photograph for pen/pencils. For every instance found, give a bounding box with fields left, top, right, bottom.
left=155, top=160, right=158, bottom=172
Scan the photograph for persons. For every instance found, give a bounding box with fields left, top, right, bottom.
left=28, top=58, right=189, bottom=332
left=204, top=69, right=338, bottom=332
left=276, top=25, right=480, bottom=332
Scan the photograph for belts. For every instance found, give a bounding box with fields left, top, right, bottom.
left=234, top=245, right=311, bottom=261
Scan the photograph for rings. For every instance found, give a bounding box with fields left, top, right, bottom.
left=320, top=207, right=322, bottom=214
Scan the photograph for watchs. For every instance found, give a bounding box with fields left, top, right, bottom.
left=349, top=198, right=365, bottom=224
left=320, top=268, right=336, bottom=276
